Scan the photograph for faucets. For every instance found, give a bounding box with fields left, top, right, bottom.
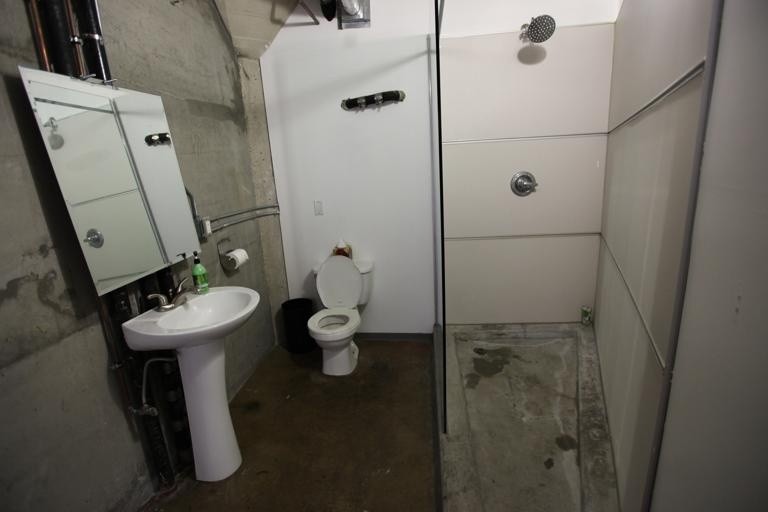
left=169, top=275, right=190, bottom=308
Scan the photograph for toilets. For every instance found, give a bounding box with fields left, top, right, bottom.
left=307, top=257, right=373, bottom=376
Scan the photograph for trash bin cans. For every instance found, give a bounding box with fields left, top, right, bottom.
left=281, top=298, right=317, bottom=349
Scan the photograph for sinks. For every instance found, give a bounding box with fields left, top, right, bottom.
left=122, top=286, right=261, bottom=351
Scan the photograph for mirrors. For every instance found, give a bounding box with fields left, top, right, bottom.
left=18, top=62, right=201, bottom=298
